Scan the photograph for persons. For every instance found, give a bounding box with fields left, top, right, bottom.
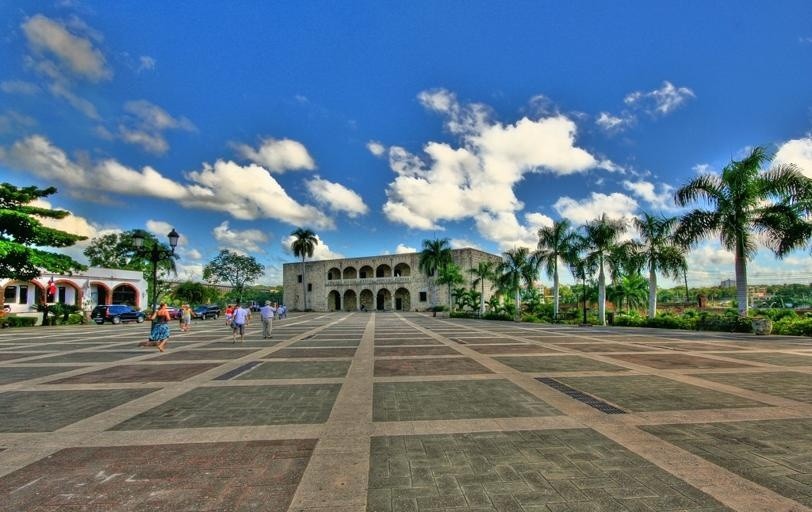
left=232, top=305, right=248, bottom=344
left=273, top=304, right=288, bottom=320
left=225, top=305, right=232, bottom=325
left=261, top=300, right=277, bottom=338
left=231, top=304, right=240, bottom=320
left=178, top=306, right=187, bottom=332
left=183, top=303, right=196, bottom=329
left=244, top=306, right=251, bottom=321
left=149, top=302, right=171, bottom=353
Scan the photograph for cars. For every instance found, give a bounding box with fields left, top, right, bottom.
left=155, top=304, right=220, bottom=321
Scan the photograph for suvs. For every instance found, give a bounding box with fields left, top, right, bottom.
left=247, top=300, right=261, bottom=313
left=91, top=304, right=145, bottom=325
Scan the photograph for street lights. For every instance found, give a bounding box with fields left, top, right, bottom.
left=131, top=226, right=179, bottom=316
left=569, top=260, right=602, bottom=328
